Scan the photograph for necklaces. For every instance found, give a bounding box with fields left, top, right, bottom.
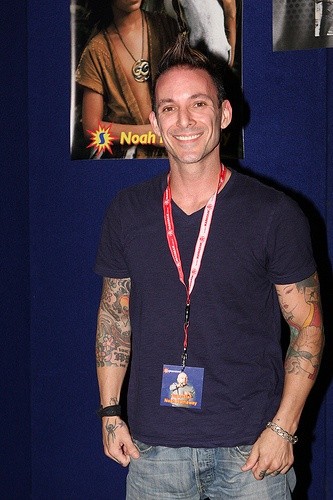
left=110, top=10, right=150, bottom=82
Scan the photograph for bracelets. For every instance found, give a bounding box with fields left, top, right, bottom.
left=267, top=421, right=298, bottom=444
left=96, top=404, right=122, bottom=422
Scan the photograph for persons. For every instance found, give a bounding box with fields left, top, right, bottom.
left=75, top=0, right=236, bottom=159
left=93, top=28, right=325, bottom=500
left=170, top=372, right=196, bottom=407
